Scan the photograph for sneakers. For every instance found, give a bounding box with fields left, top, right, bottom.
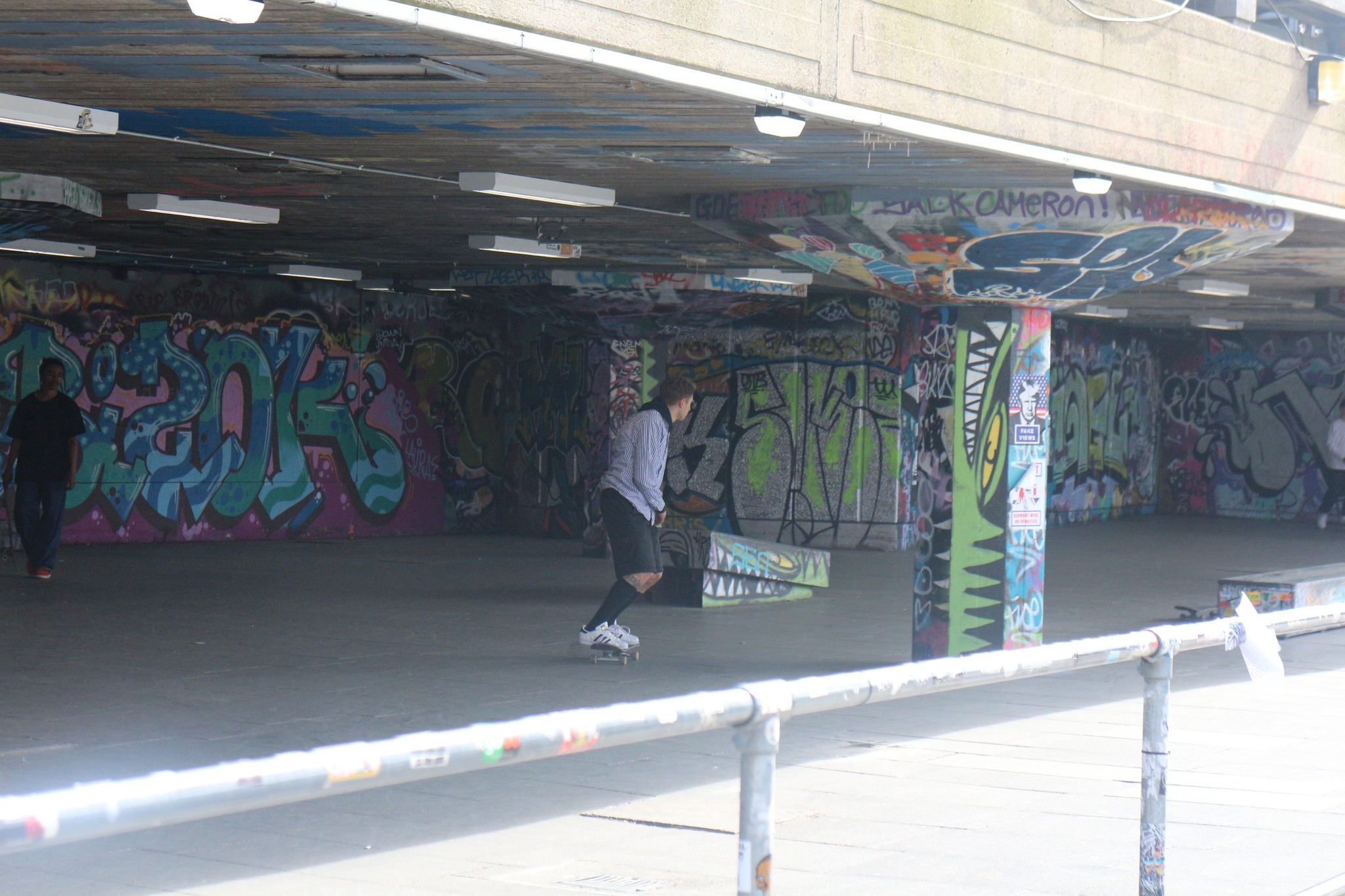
left=607, top=619, right=640, bottom=645
left=25, top=565, right=50, bottom=578
left=579, top=622, right=629, bottom=650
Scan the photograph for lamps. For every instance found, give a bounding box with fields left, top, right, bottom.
left=1191, top=317, right=1244, bottom=330
left=1308, top=54, right=1345, bottom=105
left=0, top=238, right=96, bottom=257
left=754, top=105, right=806, bottom=137
left=269, top=264, right=395, bottom=290
left=1072, top=169, right=1113, bottom=195
left=128, top=194, right=280, bottom=224
left=1178, top=278, right=1250, bottom=296
left=468, top=236, right=581, bottom=258
left=459, top=172, right=615, bottom=207
left=725, top=269, right=813, bottom=285
left=0, top=93, right=118, bottom=135
left=1073, top=304, right=1128, bottom=318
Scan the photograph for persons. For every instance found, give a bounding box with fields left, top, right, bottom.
left=580, top=377, right=696, bottom=650
left=1317, top=398, right=1345, bottom=528
left=2, top=357, right=86, bottom=579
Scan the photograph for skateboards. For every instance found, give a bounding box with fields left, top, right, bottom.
left=1174, top=605, right=1218, bottom=621
left=0, top=487, right=15, bottom=563
left=591, top=643, right=640, bottom=665
